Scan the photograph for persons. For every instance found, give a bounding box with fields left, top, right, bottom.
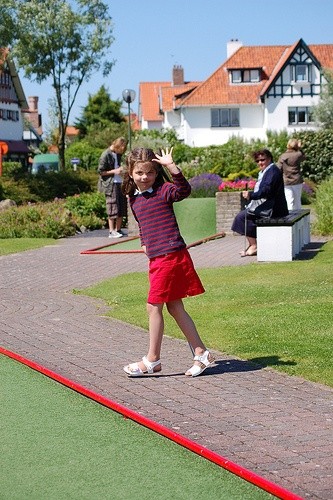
left=98, top=136, right=129, bottom=238
left=278, top=138, right=306, bottom=210
left=231, top=149, right=289, bottom=257
left=122, top=147, right=216, bottom=377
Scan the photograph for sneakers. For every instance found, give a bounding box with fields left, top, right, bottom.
left=109, top=231, right=123, bottom=238
left=118, top=231, right=128, bottom=236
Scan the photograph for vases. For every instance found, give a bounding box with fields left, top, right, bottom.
left=216, top=189, right=253, bottom=233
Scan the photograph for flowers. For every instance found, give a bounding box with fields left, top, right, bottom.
left=216, top=176, right=258, bottom=191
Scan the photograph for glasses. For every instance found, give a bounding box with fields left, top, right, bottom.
left=255, top=159, right=267, bottom=162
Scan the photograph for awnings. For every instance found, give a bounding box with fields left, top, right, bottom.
left=0, top=140, right=30, bottom=155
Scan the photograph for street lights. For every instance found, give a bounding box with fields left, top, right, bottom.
left=122, top=88, right=137, bottom=153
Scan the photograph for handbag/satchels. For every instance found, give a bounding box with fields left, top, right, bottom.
left=248, top=198, right=274, bottom=219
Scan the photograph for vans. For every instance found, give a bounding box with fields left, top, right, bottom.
left=32, top=153, right=60, bottom=178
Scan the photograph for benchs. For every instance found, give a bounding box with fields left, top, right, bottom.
left=257, top=209, right=311, bottom=262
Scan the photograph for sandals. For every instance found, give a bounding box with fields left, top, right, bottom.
left=124, top=356, right=163, bottom=376
left=186, top=351, right=216, bottom=377
